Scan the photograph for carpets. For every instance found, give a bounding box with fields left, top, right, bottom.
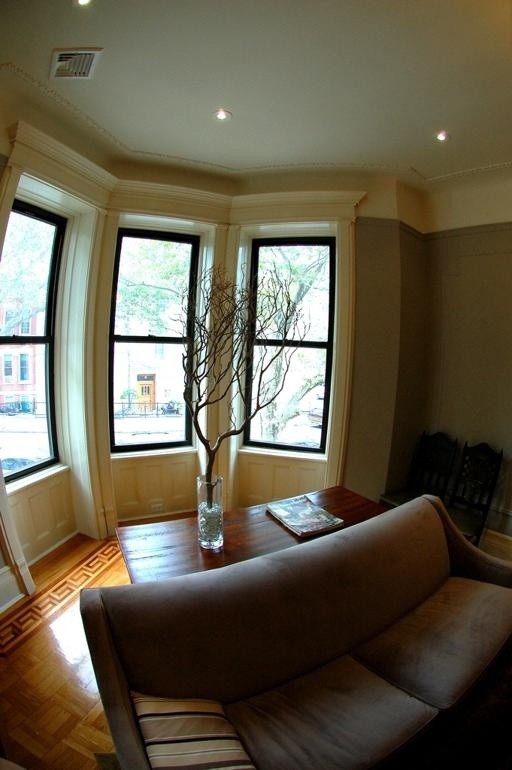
left=93, top=692, right=512, bottom=770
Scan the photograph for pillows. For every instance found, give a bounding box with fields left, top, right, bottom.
left=129, top=688, right=255, bottom=770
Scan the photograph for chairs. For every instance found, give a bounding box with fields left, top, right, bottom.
left=380, top=424, right=507, bottom=553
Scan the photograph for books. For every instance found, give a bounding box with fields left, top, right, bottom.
left=265, top=494, right=345, bottom=538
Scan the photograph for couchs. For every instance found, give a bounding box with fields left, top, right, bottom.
left=77, top=492, right=512, bottom=770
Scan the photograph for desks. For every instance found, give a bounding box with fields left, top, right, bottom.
left=112, top=482, right=388, bottom=585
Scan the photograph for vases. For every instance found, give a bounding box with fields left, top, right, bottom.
left=194, top=472, right=223, bottom=553
